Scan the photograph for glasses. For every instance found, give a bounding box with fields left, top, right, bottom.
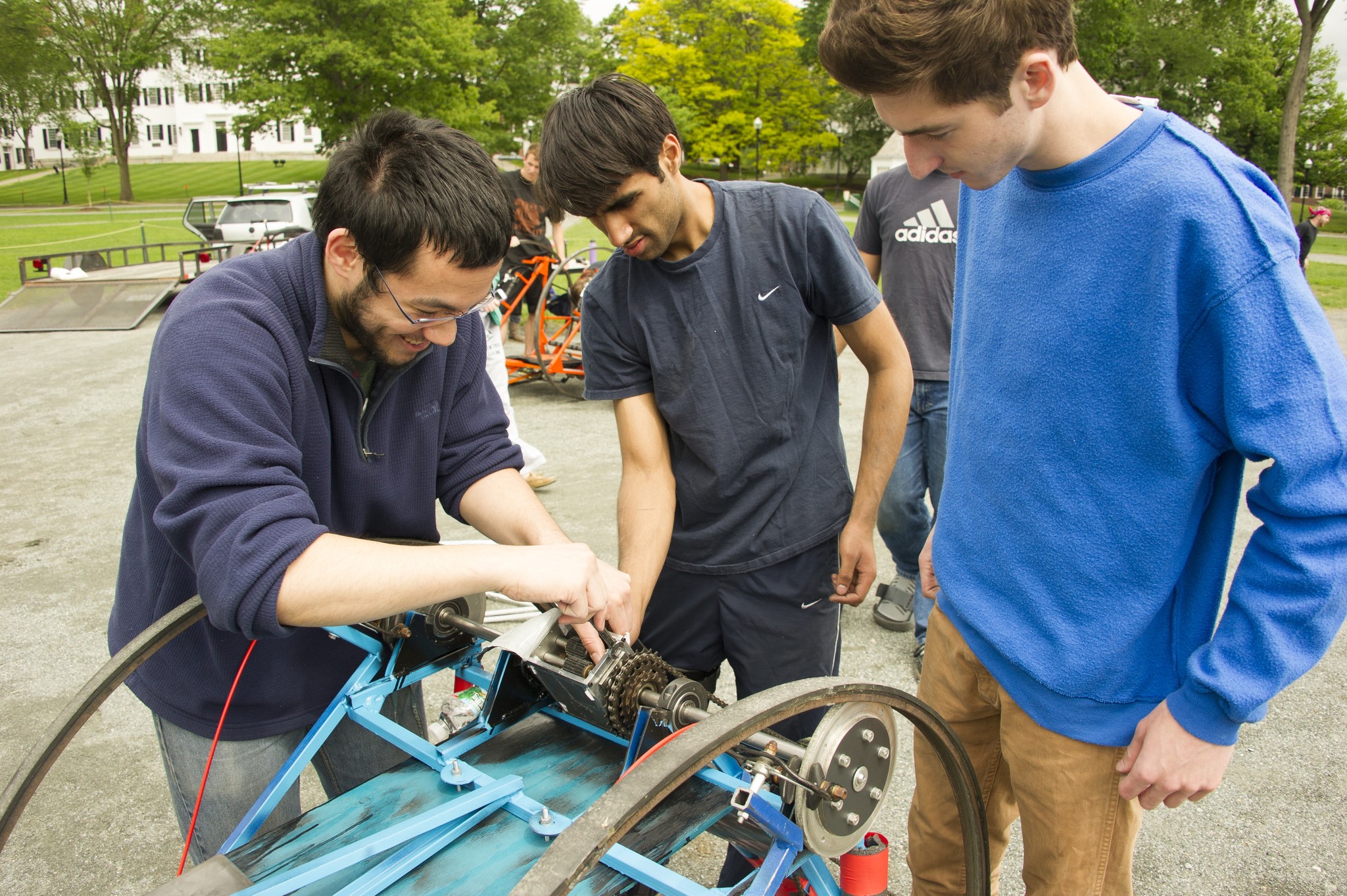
left=371, top=261, right=495, bottom=328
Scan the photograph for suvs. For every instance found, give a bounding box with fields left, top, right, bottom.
left=182, top=180, right=319, bottom=259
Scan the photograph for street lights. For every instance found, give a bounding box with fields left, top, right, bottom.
left=55, top=132, right=70, bottom=204
left=836, top=128, right=843, bottom=199
left=1298, top=158, right=1312, bottom=224
left=754, top=117, right=763, bottom=182
left=233, top=124, right=246, bottom=196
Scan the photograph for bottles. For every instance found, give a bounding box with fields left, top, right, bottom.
left=427, top=685, right=489, bottom=745
left=590, top=241, right=597, bottom=264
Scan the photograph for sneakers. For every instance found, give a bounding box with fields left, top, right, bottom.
left=915, top=646, right=930, bottom=679
left=872, top=576, right=917, bottom=629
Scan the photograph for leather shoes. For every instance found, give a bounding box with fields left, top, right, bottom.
left=527, top=474, right=557, bottom=489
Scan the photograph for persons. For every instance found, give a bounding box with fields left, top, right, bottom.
left=1295, top=204, right=1332, bottom=280
left=108, top=103, right=635, bottom=868
left=496, top=141, right=566, bottom=358
left=536, top=79, right=914, bottom=888
left=803, top=0, right=1347, bottom=896
left=831, top=154, right=963, bottom=687
